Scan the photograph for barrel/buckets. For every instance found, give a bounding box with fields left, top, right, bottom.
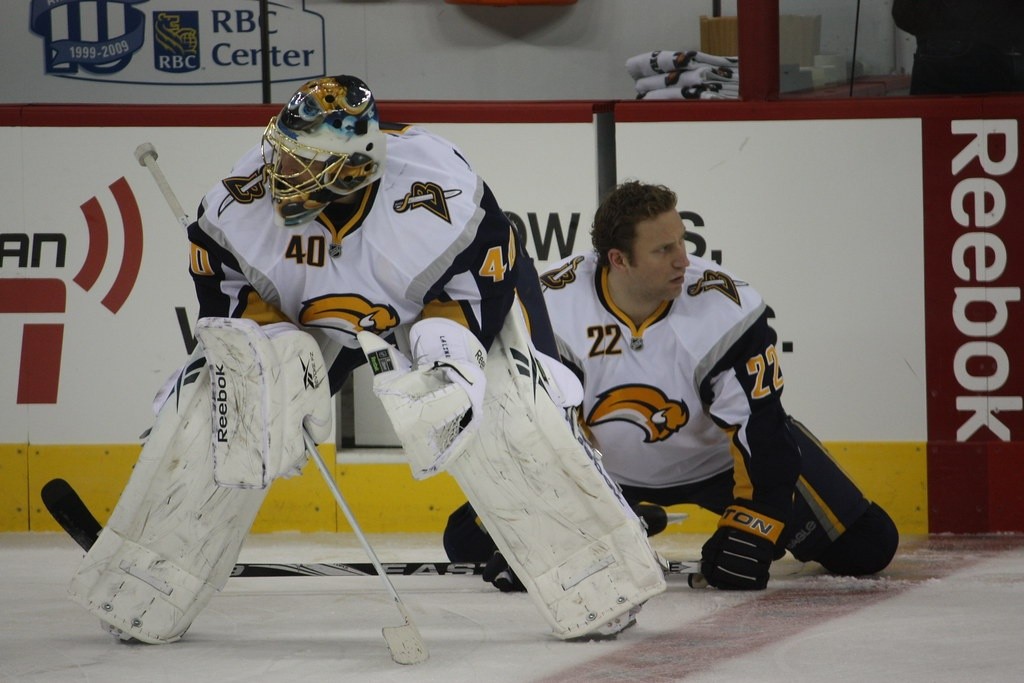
left=701, top=16, right=821, bottom=67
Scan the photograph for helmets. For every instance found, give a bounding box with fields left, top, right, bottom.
left=276, top=75, right=385, bottom=195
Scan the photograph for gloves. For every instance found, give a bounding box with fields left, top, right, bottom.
left=481, top=551, right=527, bottom=593
left=701, top=526, right=785, bottom=590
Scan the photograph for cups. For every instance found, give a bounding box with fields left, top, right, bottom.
left=812, top=65, right=838, bottom=95
left=814, top=54, right=847, bottom=84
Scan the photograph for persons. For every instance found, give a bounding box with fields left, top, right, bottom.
left=892, top=0, right=1024, bottom=95
left=76, top=75, right=668, bottom=649
left=442, top=181, right=900, bottom=594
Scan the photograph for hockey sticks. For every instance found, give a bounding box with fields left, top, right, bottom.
left=135, top=142, right=432, bottom=666
left=39, top=475, right=702, bottom=581
